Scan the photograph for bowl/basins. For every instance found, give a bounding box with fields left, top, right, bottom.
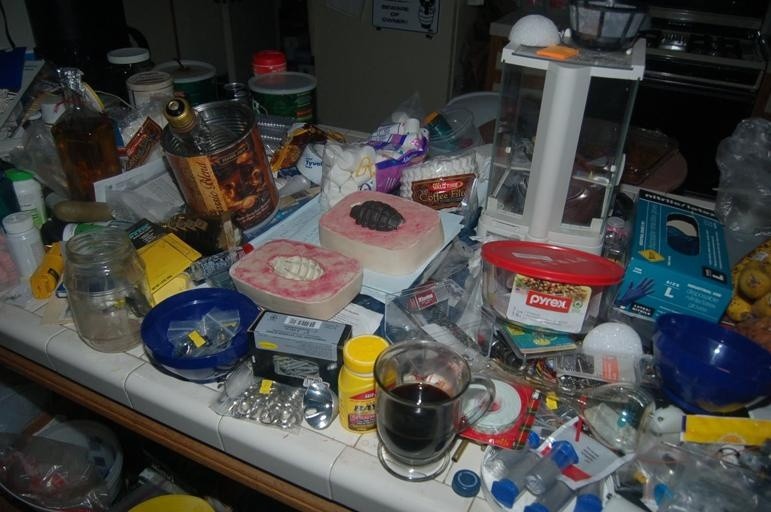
left=139, top=286, right=258, bottom=385
left=651, top=311, right=771, bottom=416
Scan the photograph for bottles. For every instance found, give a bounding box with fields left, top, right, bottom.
left=572, top=483, right=602, bottom=512
left=422, top=110, right=457, bottom=151
left=523, top=480, right=572, bottom=512
left=105, top=48, right=154, bottom=98
left=3, top=211, right=46, bottom=277
left=525, top=439, right=579, bottom=496
left=125, top=71, right=173, bottom=113
left=63, top=230, right=156, bottom=353
left=427, top=106, right=483, bottom=157
left=485, top=430, right=541, bottom=478
left=52, top=69, right=119, bottom=199
left=491, top=450, right=540, bottom=508
left=154, top=60, right=217, bottom=106
left=247, top=72, right=318, bottom=127
left=481, top=357, right=657, bottom=454
left=5, top=168, right=47, bottom=229
left=253, top=50, right=285, bottom=80
left=336, top=335, right=396, bottom=434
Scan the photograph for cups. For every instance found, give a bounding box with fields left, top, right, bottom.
left=373, top=339, right=497, bottom=483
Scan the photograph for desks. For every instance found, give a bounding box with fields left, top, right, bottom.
left=1, top=124, right=716, bottom=512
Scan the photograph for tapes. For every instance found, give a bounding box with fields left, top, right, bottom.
left=297, top=143, right=324, bottom=185
left=463, top=379, right=521, bottom=433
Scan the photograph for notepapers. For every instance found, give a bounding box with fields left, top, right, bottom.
left=539, top=43, right=576, bottom=60
